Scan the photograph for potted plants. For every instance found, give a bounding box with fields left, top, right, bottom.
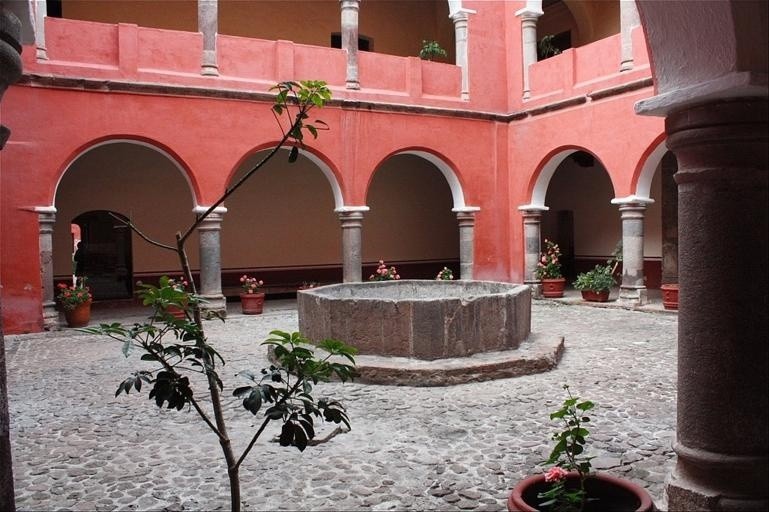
left=507, top=382, right=659, bottom=512
left=571, top=259, right=618, bottom=302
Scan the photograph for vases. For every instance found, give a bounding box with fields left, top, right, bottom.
left=63, top=298, right=93, bottom=328
left=240, top=293, right=265, bottom=314
left=541, top=279, right=567, bottom=298
left=160, top=304, right=189, bottom=321
left=660, top=283, right=679, bottom=310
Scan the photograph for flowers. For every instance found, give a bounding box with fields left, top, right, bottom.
left=434, top=266, right=454, bottom=280
left=240, top=274, right=264, bottom=294
left=368, top=259, right=401, bottom=281
left=168, top=276, right=188, bottom=292
left=298, top=280, right=321, bottom=289
left=535, top=238, right=563, bottom=280
left=56, top=283, right=94, bottom=312
